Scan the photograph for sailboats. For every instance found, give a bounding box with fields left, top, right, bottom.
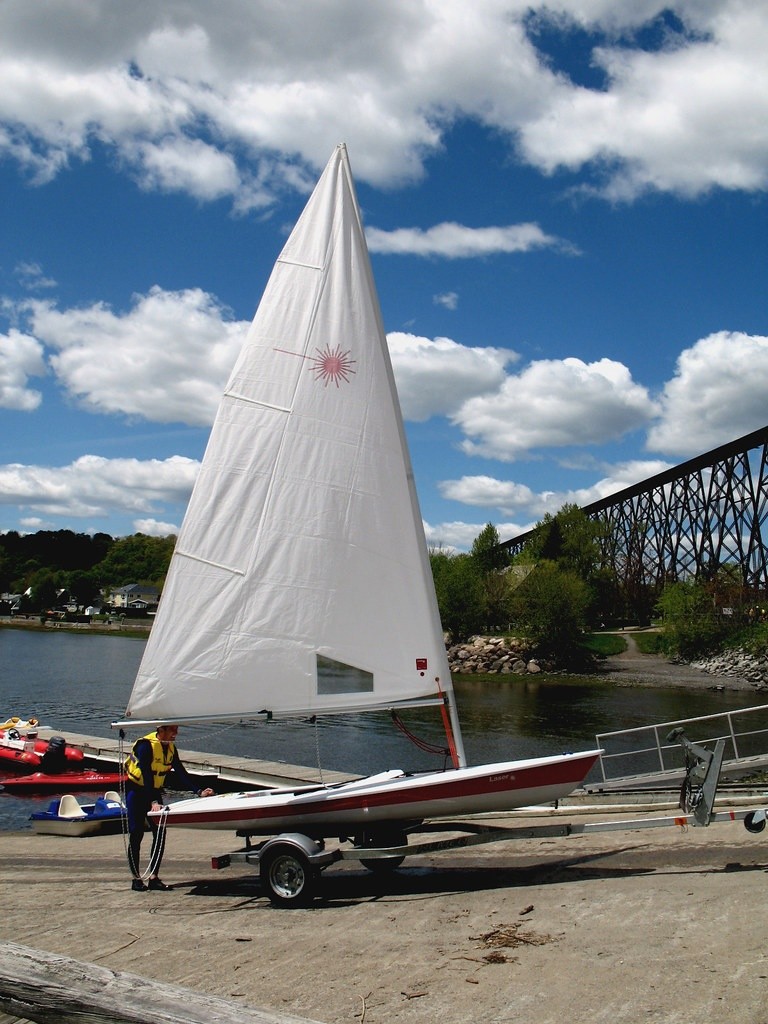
left=109, top=141, right=607, bottom=833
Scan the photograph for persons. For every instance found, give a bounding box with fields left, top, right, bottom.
left=124, top=725, right=215, bottom=892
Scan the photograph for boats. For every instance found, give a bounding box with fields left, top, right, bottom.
left=0, top=716, right=131, bottom=793
left=28, top=790, right=129, bottom=838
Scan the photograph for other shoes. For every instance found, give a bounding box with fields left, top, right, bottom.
left=132, top=878, right=148, bottom=891
left=148, top=876, right=170, bottom=890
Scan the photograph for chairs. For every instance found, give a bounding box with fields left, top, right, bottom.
left=24, top=720, right=38, bottom=727
left=5, top=717, right=23, bottom=725
left=103, top=790, right=128, bottom=815
left=58, top=794, right=88, bottom=818
